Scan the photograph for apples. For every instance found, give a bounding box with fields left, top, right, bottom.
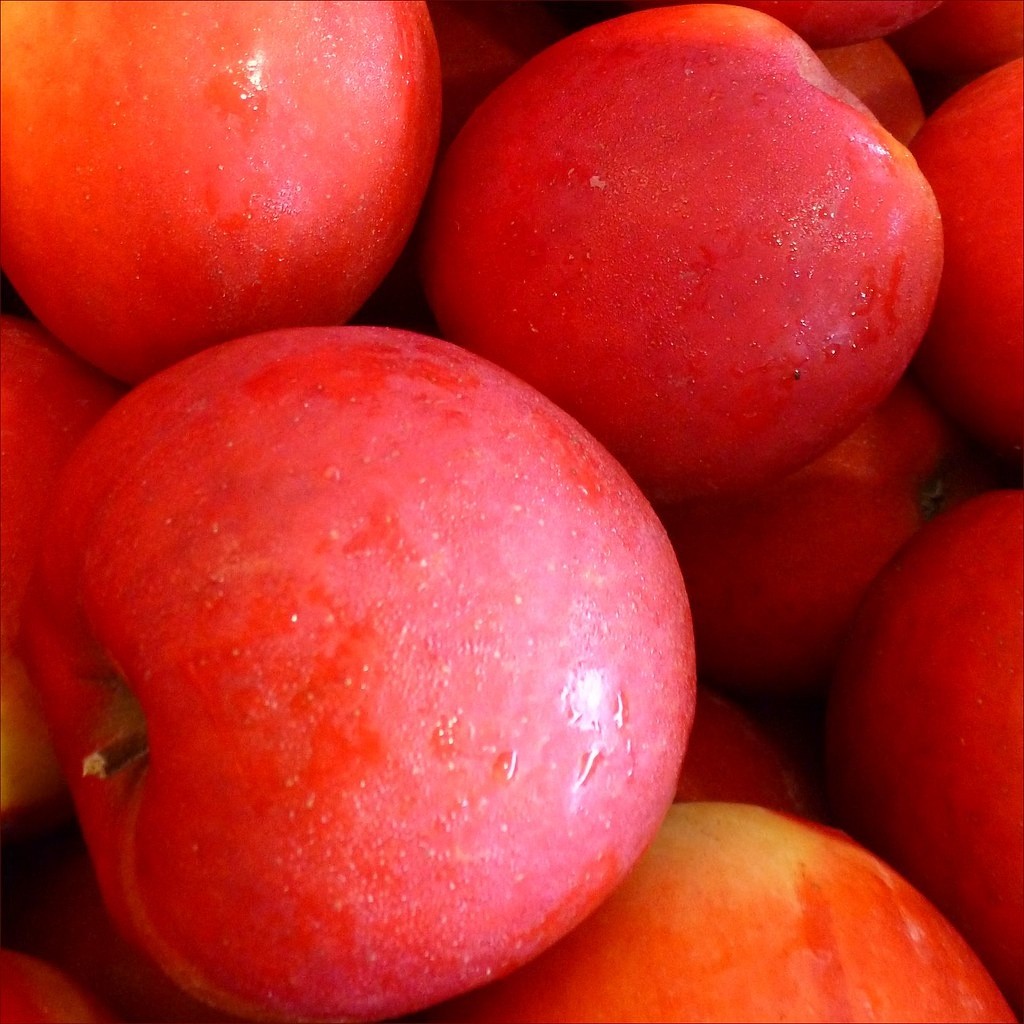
left=0, top=0, right=1024, bottom=1024
